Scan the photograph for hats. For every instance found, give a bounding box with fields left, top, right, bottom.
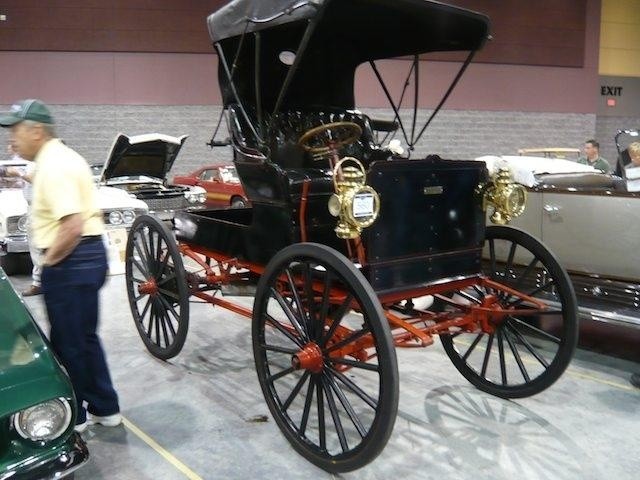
left=0, top=98, right=55, bottom=127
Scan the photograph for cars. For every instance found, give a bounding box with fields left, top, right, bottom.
left=0, top=266, right=93, bottom=480
left=89, top=131, right=207, bottom=231
left=0, top=159, right=151, bottom=276
left=481, top=146, right=640, bottom=360
left=173, top=161, right=254, bottom=211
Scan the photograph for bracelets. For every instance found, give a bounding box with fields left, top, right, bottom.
left=19, top=170, right=24, bottom=179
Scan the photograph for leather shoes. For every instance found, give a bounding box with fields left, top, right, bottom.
left=22, top=285, right=45, bottom=296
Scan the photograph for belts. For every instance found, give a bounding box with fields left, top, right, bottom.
left=78, top=235, right=102, bottom=248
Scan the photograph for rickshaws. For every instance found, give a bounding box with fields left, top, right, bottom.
left=126, top=3, right=584, bottom=475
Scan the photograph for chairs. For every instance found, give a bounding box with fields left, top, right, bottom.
left=229, top=104, right=385, bottom=203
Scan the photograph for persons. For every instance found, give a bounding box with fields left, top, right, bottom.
left=0, top=159, right=43, bottom=297
left=573, top=139, right=611, bottom=175
left=623, top=141, right=640, bottom=169
left=1, top=98, right=122, bottom=434
left=6, top=143, right=21, bottom=162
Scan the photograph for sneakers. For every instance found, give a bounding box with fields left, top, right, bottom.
left=73, top=408, right=122, bottom=433
